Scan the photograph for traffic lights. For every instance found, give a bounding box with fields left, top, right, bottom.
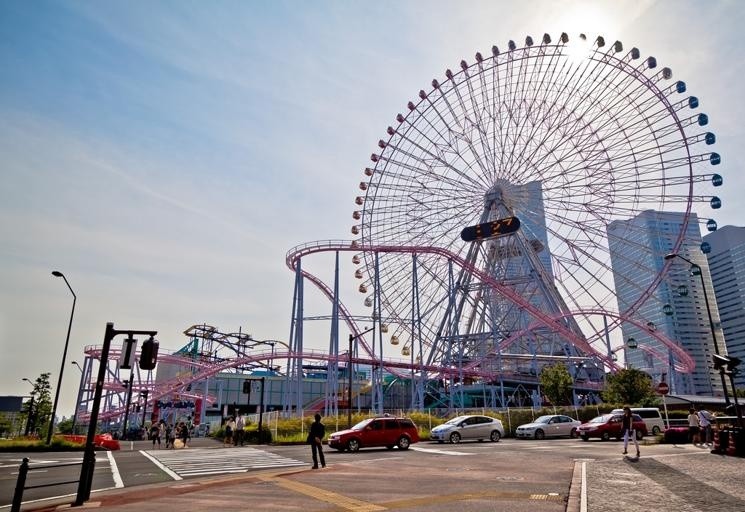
left=139, top=391, right=146, bottom=398
left=140, top=339, right=159, bottom=370
left=136, top=405, right=141, bottom=412
left=243, top=381, right=249, bottom=393
left=122, top=380, right=128, bottom=388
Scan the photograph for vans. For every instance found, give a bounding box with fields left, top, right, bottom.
left=610, top=408, right=666, bottom=435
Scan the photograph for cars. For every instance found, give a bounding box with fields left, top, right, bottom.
left=576, top=414, right=648, bottom=442
left=328, top=417, right=421, bottom=452
left=516, top=415, right=582, bottom=440
left=430, top=415, right=506, bottom=444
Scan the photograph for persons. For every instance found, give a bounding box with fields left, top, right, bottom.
left=620, top=407, right=640, bottom=459
left=110, top=414, right=246, bottom=450
left=310, top=413, right=325, bottom=469
left=687, top=409, right=713, bottom=448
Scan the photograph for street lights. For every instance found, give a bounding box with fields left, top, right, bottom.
left=664, top=253, right=731, bottom=407
left=23, top=271, right=85, bottom=444
